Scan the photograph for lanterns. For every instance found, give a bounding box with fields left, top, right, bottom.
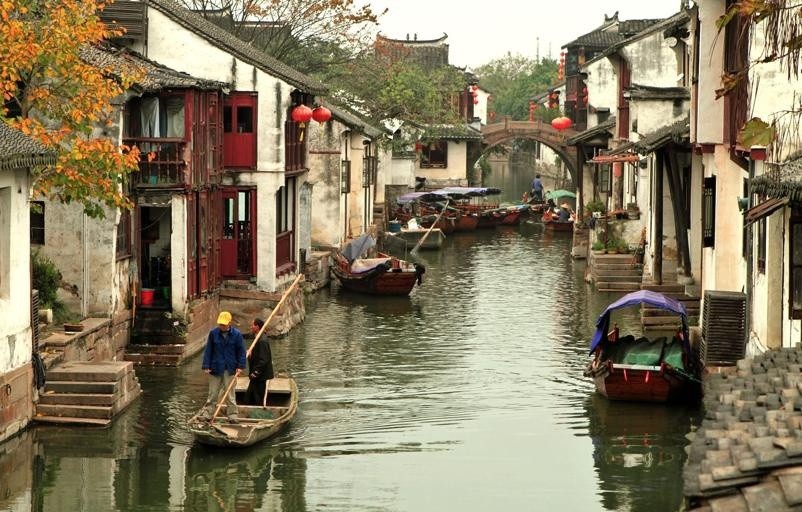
left=292, top=104, right=312, bottom=141
left=471, top=85, right=478, bottom=106
left=312, top=106, right=331, bottom=132
left=529, top=52, right=588, bottom=130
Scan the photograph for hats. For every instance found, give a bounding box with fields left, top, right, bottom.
left=217, top=311, right=232, bottom=325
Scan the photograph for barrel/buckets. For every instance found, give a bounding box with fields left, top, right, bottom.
left=407, top=217, right=418, bottom=230
left=388, top=220, right=401, bottom=232
left=628, top=206, right=640, bottom=220
left=139, top=286, right=156, bottom=305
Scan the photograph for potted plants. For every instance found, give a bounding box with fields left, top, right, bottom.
left=586, top=201, right=605, bottom=218
left=591, top=238, right=629, bottom=254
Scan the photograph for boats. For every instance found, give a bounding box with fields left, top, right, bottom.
left=390, top=186, right=576, bottom=250
left=187, top=372, right=299, bottom=447
left=583, top=289, right=692, bottom=402
left=328, top=233, right=425, bottom=297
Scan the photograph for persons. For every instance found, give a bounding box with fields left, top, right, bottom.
left=532, top=174, right=543, bottom=202
left=201, top=311, right=246, bottom=423
left=246, top=318, right=274, bottom=406
left=557, top=204, right=570, bottom=221
